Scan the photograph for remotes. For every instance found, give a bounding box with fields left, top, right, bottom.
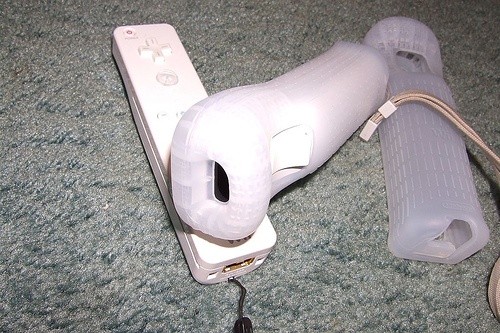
left=110, top=21, right=278, bottom=285
